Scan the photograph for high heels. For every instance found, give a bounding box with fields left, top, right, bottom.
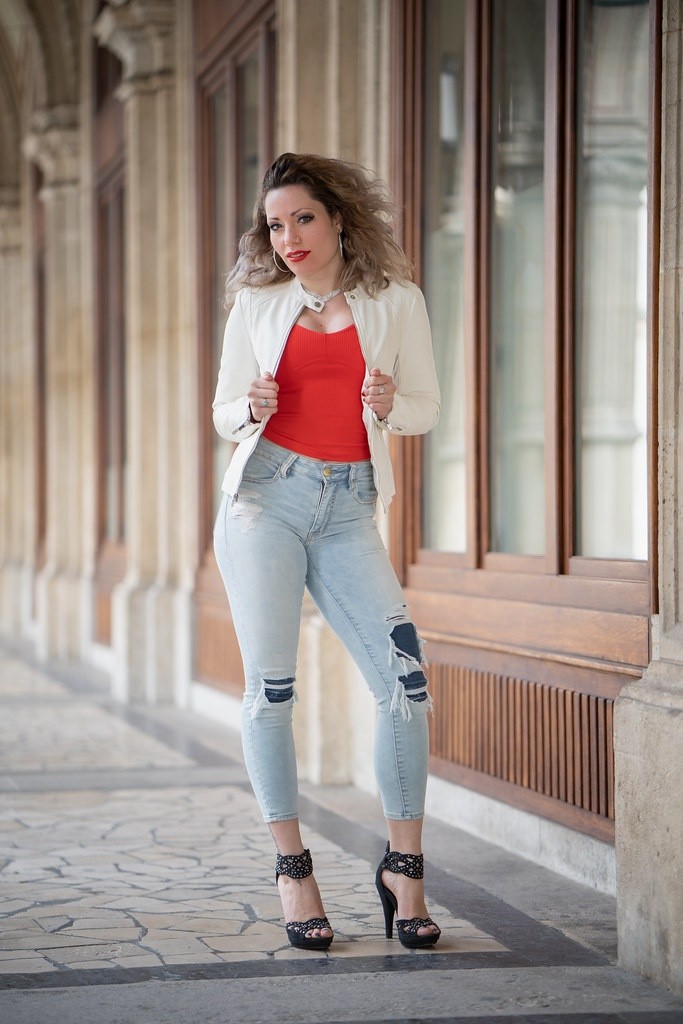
left=375, top=841, right=441, bottom=948
left=274, top=849, right=333, bottom=949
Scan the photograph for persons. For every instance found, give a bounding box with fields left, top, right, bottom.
left=214, top=153, right=440, bottom=948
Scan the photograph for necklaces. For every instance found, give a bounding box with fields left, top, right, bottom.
left=302, top=286, right=344, bottom=303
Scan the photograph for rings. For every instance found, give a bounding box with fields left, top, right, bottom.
left=262, top=398, right=268, bottom=407
left=378, top=385, right=385, bottom=395
